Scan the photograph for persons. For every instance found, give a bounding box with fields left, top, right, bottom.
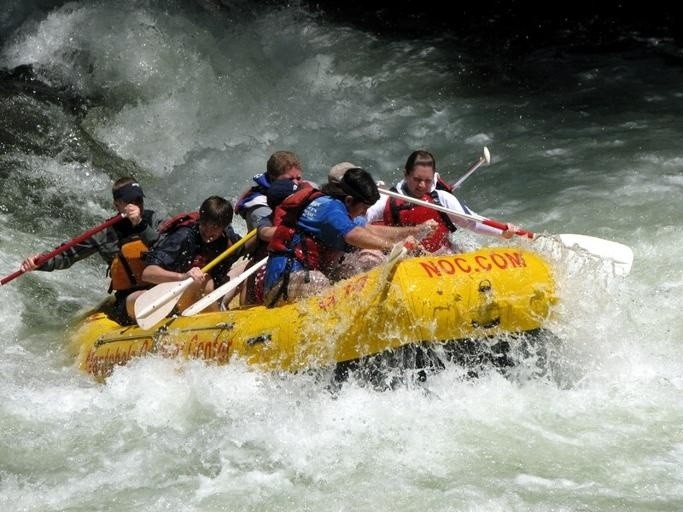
left=235, top=150, right=519, bottom=309
left=20, top=177, right=163, bottom=327
left=141, top=196, right=257, bottom=316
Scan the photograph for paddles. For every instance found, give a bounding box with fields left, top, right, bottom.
left=377, top=188, right=633, bottom=277
left=452, top=145, right=490, bottom=193
left=389, top=226, right=431, bottom=266
left=133, top=228, right=268, bottom=332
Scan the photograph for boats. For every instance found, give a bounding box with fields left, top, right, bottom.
left=55, top=244, right=563, bottom=385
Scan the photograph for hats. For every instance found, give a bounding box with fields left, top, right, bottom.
left=113, top=183, right=145, bottom=202
left=328, top=161, right=362, bottom=183
